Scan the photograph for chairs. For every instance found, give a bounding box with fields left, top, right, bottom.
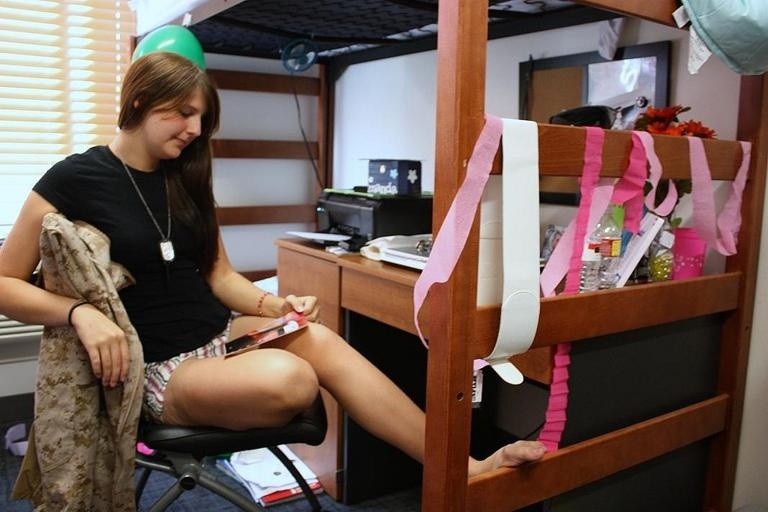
left=40, top=214, right=328, bottom=512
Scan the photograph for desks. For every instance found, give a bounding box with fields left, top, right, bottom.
left=275, top=235, right=742, bottom=512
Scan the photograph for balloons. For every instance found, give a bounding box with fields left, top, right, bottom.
left=128, top=27, right=208, bottom=72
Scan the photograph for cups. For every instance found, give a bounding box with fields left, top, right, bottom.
left=672, top=226, right=706, bottom=280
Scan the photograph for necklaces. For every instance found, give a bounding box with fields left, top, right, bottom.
left=114, top=148, right=179, bottom=262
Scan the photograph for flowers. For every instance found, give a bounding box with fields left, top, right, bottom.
left=635, top=102, right=717, bottom=228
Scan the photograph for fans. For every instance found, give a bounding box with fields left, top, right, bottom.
left=274, top=38, right=319, bottom=74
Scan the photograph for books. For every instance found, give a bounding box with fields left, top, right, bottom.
left=214, top=440, right=325, bottom=506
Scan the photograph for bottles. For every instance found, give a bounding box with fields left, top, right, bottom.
left=580, top=205, right=621, bottom=292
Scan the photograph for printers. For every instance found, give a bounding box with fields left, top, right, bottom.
left=284, top=188, right=433, bottom=253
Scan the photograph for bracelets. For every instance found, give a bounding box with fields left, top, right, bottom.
left=257, top=291, right=275, bottom=316
left=67, top=300, right=94, bottom=327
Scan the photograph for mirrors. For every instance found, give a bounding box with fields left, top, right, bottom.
left=519, top=41, right=675, bottom=207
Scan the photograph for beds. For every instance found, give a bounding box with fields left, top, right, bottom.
left=129, top=0, right=768, bottom=512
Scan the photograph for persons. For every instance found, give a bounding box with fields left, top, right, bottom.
left=0, top=52, right=552, bottom=480
